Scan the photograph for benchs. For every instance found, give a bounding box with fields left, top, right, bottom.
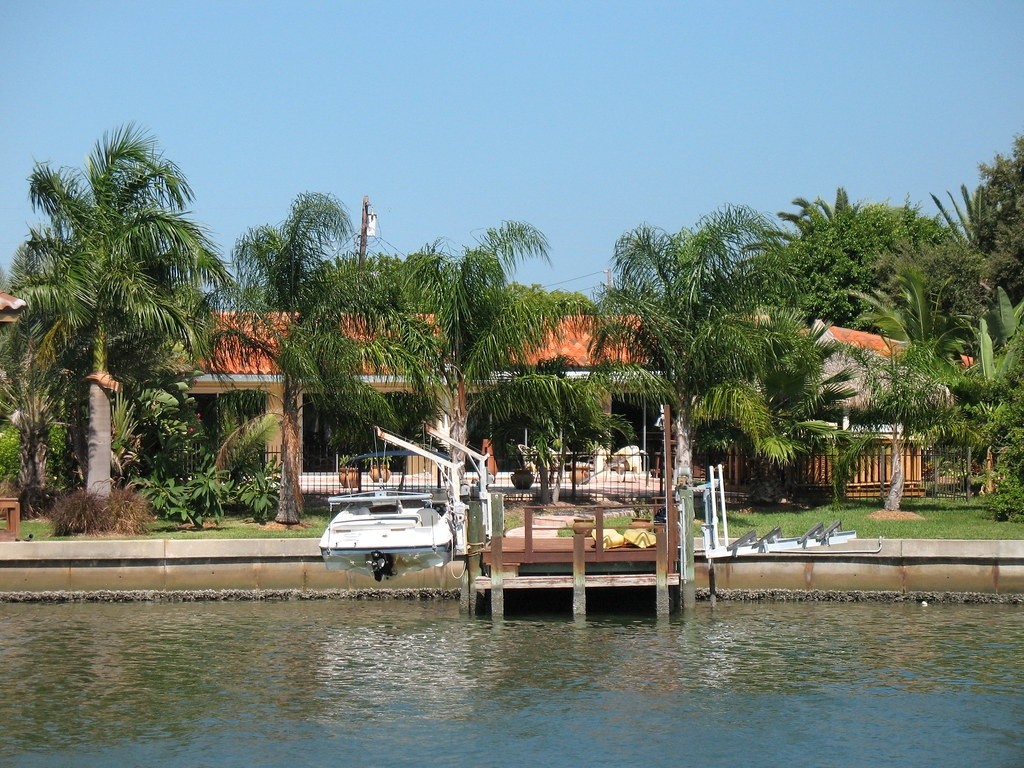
left=0, top=498, right=21, bottom=535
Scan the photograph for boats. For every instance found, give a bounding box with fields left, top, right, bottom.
left=319, top=484, right=457, bottom=577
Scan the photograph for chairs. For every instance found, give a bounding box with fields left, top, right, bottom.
left=604, top=446, right=642, bottom=483
left=517, top=444, right=565, bottom=484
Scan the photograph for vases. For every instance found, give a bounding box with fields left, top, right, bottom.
left=572, top=519, right=594, bottom=538
left=569, top=466, right=590, bottom=485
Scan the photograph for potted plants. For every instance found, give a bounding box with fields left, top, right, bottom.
left=362, top=454, right=392, bottom=483
left=505, top=443, right=535, bottom=490
left=630, top=500, right=652, bottom=533
left=338, top=453, right=359, bottom=488
